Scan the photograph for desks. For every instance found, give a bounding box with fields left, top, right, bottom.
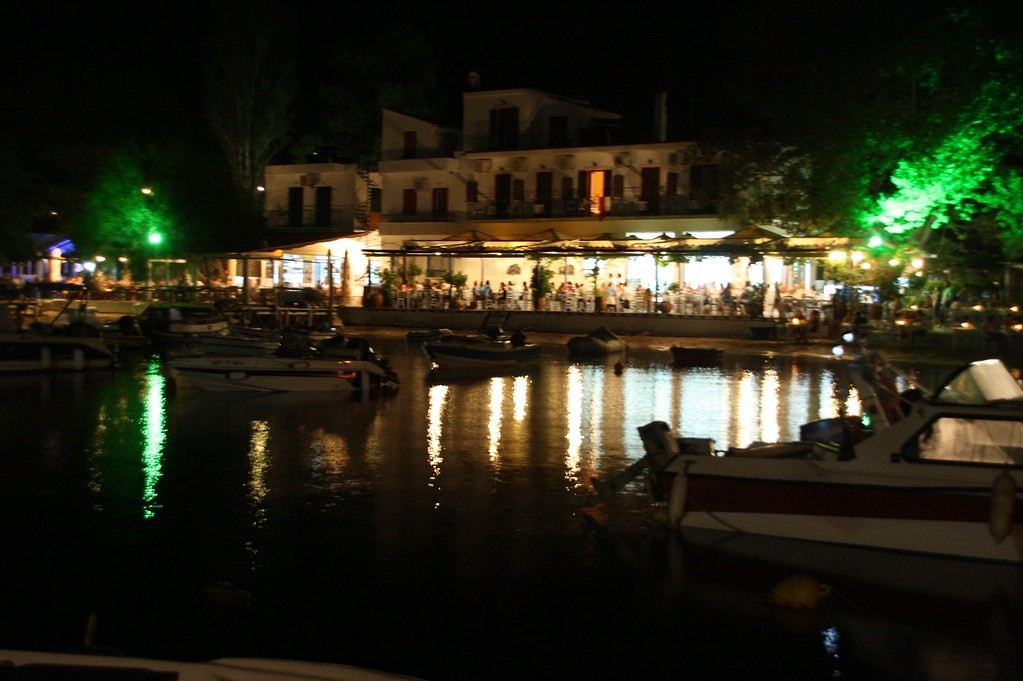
left=926, top=328, right=953, bottom=349
left=872, top=328, right=888, bottom=349
left=952, top=327, right=979, bottom=350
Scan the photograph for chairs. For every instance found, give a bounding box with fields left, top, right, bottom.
left=849, top=307, right=1023, bottom=354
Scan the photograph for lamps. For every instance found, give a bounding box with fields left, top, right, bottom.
left=256, top=181, right=266, bottom=193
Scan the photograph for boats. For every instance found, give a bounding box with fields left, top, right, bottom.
left=577, top=323, right=1023, bottom=569
left=403, top=329, right=451, bottom=343
left=669, top=346, right=725, bottom=364
left=566, top=324, right=628, bottom=354
left=419, top=329, right=543, bottom=369
left=0, top=280, right=402, bottom=394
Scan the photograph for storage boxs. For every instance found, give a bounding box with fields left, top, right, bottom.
left=801, top=416, right=863, bottom=449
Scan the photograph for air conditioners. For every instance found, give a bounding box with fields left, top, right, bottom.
left=471, top=157, right=492, bottom=173
left=510, top=157, right=529, bottom=171
left=300, top=175, right=318, bottom=186
left=414, top=178, right=430, bottom=192
left=666, top=151, right=689, bottom=165
left=554, top=155, right=575, bottom=170
left=614, top=153, right=631, bottom=167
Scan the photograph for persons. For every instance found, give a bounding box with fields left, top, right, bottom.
left=252, top=278, right=262, bottom=300
left=53, top=269, right=232, bottom=297
left=315, top=280, right=324, bottom=290
left=400, top=273, right=974, bottom=347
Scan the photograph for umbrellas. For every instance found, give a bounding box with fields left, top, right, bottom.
left=720, top=223, right=792, bottom=241
left=440, top=229, right=503, bottom=243
left=519, top=228, right=578, bottom=242
left=580, top=232, right=700, bottom=248
